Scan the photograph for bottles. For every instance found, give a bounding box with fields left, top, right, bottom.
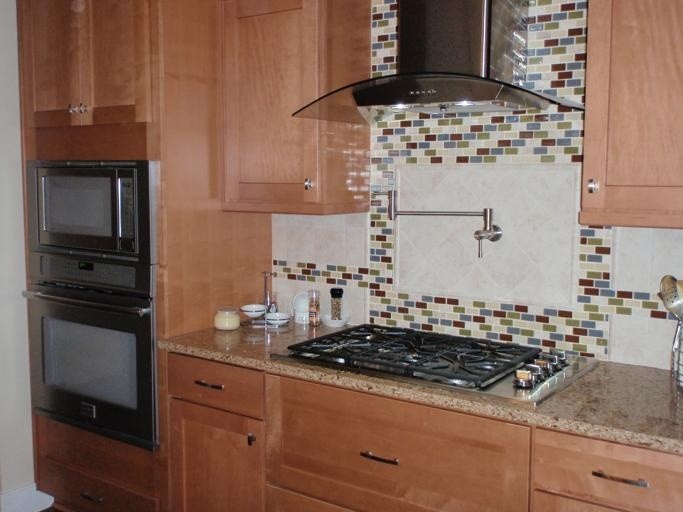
left=328, top=287, right=343, bottom=320
left=212, top=307, right=240, bottom=331
left=307, top=290, right=321, bottom=326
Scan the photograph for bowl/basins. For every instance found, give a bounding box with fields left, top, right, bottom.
left=238, top=304, right=268, bottom=319
left=263, top=312, right=290, bottom=326
left=321, top=315, right=350, bottom=327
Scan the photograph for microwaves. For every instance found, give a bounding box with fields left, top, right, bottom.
left=35, top=167, right=140, bottom=256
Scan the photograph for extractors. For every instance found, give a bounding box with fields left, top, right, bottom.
left=290, top=0, right=584, bottom=127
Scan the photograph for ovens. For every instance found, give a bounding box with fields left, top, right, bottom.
left=20, top=283, right=161, bottom=453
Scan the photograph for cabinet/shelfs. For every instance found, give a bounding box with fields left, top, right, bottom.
left=217, top=0, right=370, bottom=217
left=577, top=0, right=682, bottom=232
left=14, top=0, right=214, bottom=128
left=29, top=413, right=160, bottom=509
left=156, top=314, right=683, bottom=512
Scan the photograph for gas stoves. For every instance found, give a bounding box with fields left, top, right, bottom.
left=269, top=323, right=601, bottom=409
